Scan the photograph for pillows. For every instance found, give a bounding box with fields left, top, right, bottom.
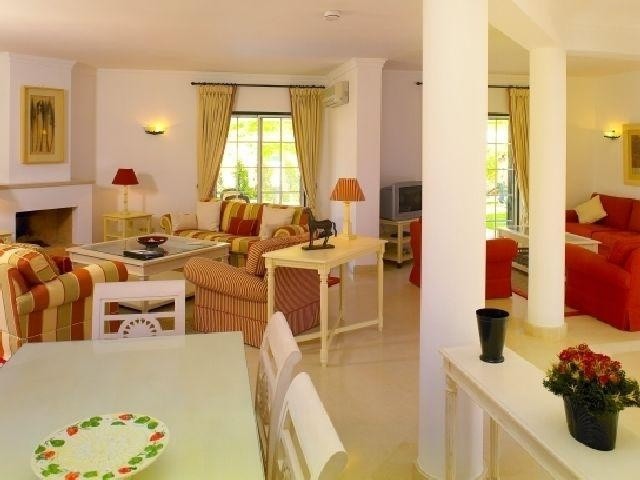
left=169, top=203, right=294, bottom=238
left=575, top=195, right=608, bottom=225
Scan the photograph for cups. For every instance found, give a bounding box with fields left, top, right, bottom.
left=476, top=308, right=509, bottom=364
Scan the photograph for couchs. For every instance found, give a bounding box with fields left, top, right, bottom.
left=566, top=242, right=640, bottom=332
left=159, top=198, right=311, bottom=269
left=410, top=217, right=517, bottom=298
left=1, top=240, right=128, bottom=365
left=566, top=192, right=640, bottom=267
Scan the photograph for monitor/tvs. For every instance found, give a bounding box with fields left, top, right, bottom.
left=380, top=180, right=422, bottom=222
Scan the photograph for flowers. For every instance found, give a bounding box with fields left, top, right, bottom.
left=542, top=343, right=640, bottom=409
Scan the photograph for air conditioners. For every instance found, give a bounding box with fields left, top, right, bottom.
left=319, top=82, right=349, bottom=108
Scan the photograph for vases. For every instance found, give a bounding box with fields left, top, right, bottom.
left=622, top=123, right=640, bottom=186
left=563, top=391, right=618, bottom=451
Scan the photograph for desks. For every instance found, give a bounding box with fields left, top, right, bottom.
left=262, top=235, right=388, bottom=368
left=0, top=331, right=266, bottom=479
left=100, top=213, right=152, bottom=241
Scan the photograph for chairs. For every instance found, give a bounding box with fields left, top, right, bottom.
left=184, top=232, right=319, bottom=349
left=253, top=311, right=349, bottom=479
left=92, top=281, right=186, bottom=339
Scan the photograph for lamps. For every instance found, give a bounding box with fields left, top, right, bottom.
left=330, top=177, right=366, bottom=239
left=111, top=169, right=137, bottom=214
left=144, top=125, right=167, bottom=135
left=604, top=130, right=621, bottom=140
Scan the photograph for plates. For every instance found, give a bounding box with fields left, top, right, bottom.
left=30, top=412, right=168, bottom=479
left=137, top=236, right=167, bottom=245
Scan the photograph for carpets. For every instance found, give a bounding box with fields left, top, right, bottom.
left=510, top=267, right=587, bottom=317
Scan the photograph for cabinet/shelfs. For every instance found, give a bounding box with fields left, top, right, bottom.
left=380, top=217, right=419, bottom=268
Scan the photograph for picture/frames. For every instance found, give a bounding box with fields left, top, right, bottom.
left=21, top=85, right=65, bottom=162
